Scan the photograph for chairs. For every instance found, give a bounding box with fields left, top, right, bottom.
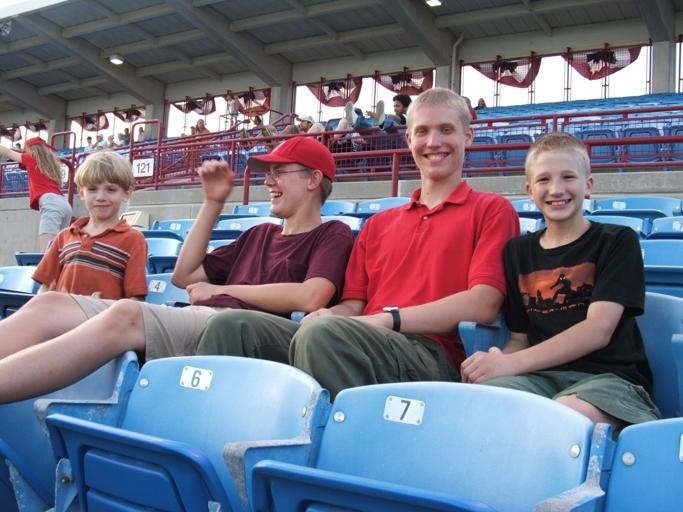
left=0, top=198, right=683, bottom=512
left=0, top=93, right=681, bottom=196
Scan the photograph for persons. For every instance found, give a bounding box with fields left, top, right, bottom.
left=0, top=133, right=355, bottom=404
left=195, top=84, right=522, bottom=405
left=0, top=137, right=73, bottom=254
left=459, top=132, right=664, bottom=431
left=29, top=149, right=149, bottom=304
left=15, top=143, right=21, bottom=149
left=474, top=97, right=486, bottom=110
left=461, top=96, right=476, bottom=121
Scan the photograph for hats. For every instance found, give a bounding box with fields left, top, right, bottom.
left=295, top=115, right=314, bottom=124
left=24, top=136, right=44, bottom=149
left=246, top=137, right=335, bottom=182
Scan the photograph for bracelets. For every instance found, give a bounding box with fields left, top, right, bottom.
left=381, top=305, right=401, bottom=332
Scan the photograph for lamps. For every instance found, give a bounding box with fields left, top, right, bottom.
left=109, top=54, right=125, bottom=65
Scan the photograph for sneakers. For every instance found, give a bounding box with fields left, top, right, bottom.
left=262, top=125, right=274, bottom=144
left=239, top=128, right=249, bottom=145
left=372, top=99, right=386, bottom=127
left=344, top=102, right=360, bottom=126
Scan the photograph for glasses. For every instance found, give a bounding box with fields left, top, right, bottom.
left=265, top=169, right=309, bottom=180
